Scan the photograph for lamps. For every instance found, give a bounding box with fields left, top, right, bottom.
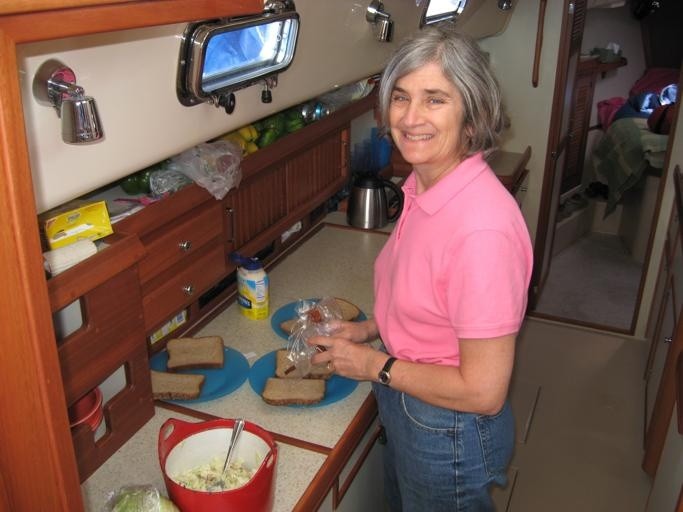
left=33, top=58, right=102, bottom=144
left=366, top=0, right=392, bottom=42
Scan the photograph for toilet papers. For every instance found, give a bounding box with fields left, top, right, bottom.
left=41, top=240, right=97, bottom=278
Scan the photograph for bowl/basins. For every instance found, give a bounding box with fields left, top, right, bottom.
left=70, top=387, right=103, bottom=434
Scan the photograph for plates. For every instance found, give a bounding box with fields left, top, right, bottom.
left=147, top=296, right=368, bottom=409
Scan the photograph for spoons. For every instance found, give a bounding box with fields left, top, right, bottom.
left=207, top=418, right=246, bottom=493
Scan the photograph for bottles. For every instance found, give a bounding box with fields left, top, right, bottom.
left=235, top=255, right=270, bottom=321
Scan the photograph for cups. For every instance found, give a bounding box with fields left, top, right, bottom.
left=353, top=142, right=380, bottom=175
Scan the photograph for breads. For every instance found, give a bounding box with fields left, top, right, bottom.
left=165, top=335, right=225, bottom=371
left=328, top=296, right=360, bottom=318
left=149, top=369, right=204, bottom=398
left=281, top=316, right=299, bottom=337
left=275, top=349, right=332, bottom=378
left=261, top=378, right=326, bottom=405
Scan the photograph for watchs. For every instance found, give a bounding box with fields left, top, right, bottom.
left=377, top=357, right=398, bottom=388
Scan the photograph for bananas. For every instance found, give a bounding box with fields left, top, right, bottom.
left=220, top=125, right=259, bottom=159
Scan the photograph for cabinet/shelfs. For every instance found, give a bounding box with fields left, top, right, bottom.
left=36, top=119, right=394, bottom=485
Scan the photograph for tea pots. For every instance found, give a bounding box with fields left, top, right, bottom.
left=347, top=170, right=403, bottom=231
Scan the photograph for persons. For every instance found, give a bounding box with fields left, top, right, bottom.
left=306, top=27, right=534, bottom=512
